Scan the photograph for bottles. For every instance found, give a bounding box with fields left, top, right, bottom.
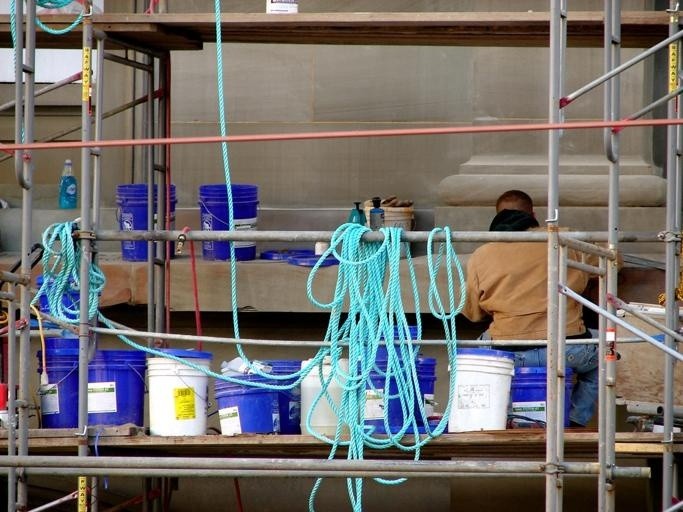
left=369, top=207, right=383, bottom=232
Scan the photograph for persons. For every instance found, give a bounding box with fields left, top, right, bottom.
left=459, top=210, right=625, bottom=430
left=497, top=188, right=534, bottom=217
left=648, top=250, right=683, bottom=512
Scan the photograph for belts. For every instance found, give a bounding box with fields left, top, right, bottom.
left=490, top=345, right=546, bottom=351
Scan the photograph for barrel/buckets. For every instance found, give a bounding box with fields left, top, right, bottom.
left=356, top=359, right=418, bottom=439
left=198, top=185, right=259, bottom=261
left=511, top=367, right=571, bottom=432
left=88, top=348, right=147, bottom=426
left=139, top=355, right=209, bottom=438
left=113, top=181, right=173, bottom=260
left=35, top=337, right=77, bottom=428
left=31, top=274, right=83, bottom=327
left=447, top=348, right=511, bottom=433
left=303, top=357, right=353, bottom=438
left=411, top=352, right=439, bottom=420
left=213, top=373, right=280, bottom=435
left=365, top=199, right=416, bottom=259
left=363, top=322, right=418, bottom=362
left=253, top=359, right=302, bottom=436
